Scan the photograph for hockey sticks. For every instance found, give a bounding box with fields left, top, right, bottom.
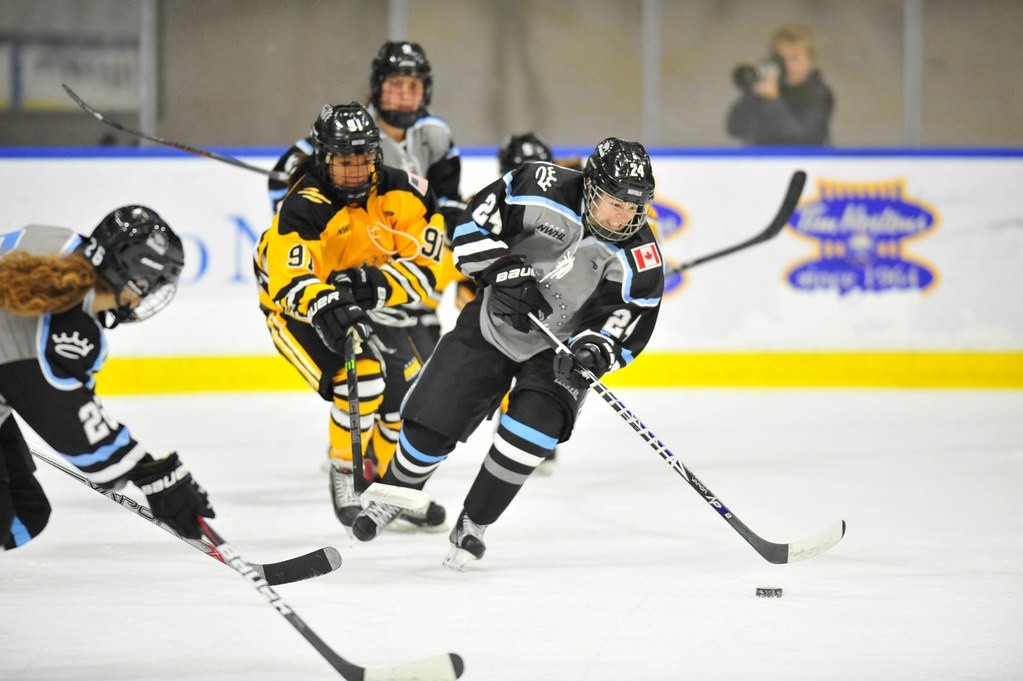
left=61, top=81, right=289, bottom=182
left=345, top=332, right=439, bottom=515
left=527, top=310, right=847, bottom=565
left=663, top=170, right=807, bottom=277
left=196, top=514, right=465, bottom=680
left=22, top=437, right=343, bottom=586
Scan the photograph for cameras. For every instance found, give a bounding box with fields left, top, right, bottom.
left=732, top=53, right=786, bottom=88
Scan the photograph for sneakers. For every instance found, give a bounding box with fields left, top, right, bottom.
left=442, top=506, right=490, bottom=573
left=399, top=490, right=448, bottom=533
left=351, top=481, right=405, bottom=542
left=327, top=463, right=364, bottom=527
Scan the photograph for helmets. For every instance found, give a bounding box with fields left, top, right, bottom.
left=497, top=132, right=554, bottom=177
left=368, top=40, right=433, bottom=129
left=75, top=206, right=185, bottom=330
left=310, top=102, right=384, bottom=204
left=583, top=137, right=657, bottom=243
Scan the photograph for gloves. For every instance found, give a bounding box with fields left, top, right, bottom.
left=307, top=289, right=376, bottom=357
left=327, top=265, right=391, bottom=312
left=127, top=452, right=216, bottom=540
left=552, top=342, right=608, bottom=390
left=482, top=254, right=553, bottom=334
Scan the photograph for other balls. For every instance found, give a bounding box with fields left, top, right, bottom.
left=755, top=586, right=782, bottom=598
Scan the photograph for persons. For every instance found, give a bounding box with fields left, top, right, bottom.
left=251, top=102, right=446, bottom=527
left=728, top=26, right=833, bottom=145
left=0, top=205, right=215, bottom=552
left=351, top=131, right=681, bottom=558
left=268, top=39, right=558, bottom=479
